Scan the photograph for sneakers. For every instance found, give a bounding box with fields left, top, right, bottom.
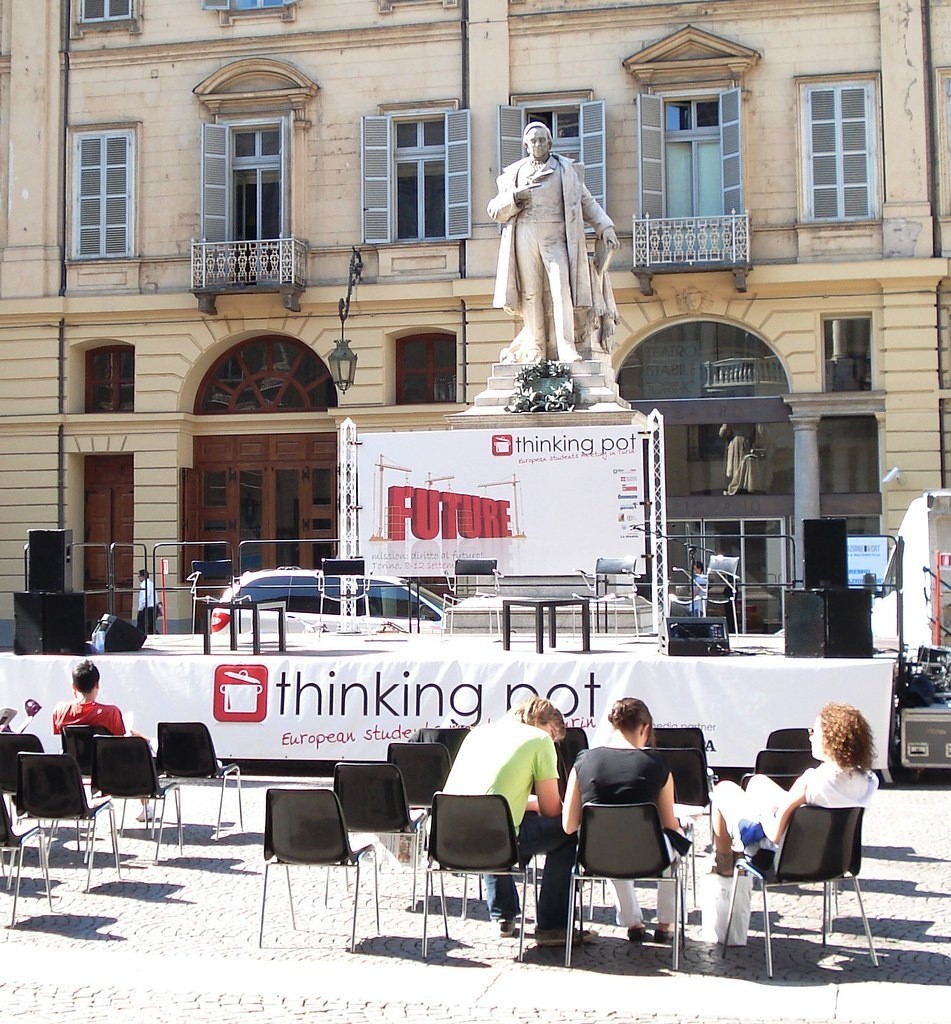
left=498, top=917, right=515, bottom=936
left=534, top=925, right=598, bottom=945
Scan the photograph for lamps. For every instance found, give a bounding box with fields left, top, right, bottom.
left=328, top=246, right=363, bottom=395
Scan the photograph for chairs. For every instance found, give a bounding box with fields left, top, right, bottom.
left=258, top=727, right=879, bottom=979
left=0, top=723, right=244, bottom=928
left=191, top=559, right=242, bottom=634
left=317, top=557, right=371, bottom=632
left=669, top=554, right=741, bottom=635
left=572, top=558, right=638, bottom=635
left=442, top=558, right=506, bottom=637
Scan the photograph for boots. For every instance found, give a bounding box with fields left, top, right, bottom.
left=732, top=849, right=746, bottom=877
left=711, top=852, right=732, bottom=877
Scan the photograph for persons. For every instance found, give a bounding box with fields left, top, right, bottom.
left=689, top=561, right=708, bottom=617
left=137, top=568, right=160, bottom=634
left=563, top=698, right=693, bottom=941
left=51, top=661, right=161, bottom=821
left=486, top=122, right=620, bottom=360
left=443, top=699, right=594, bottom=946
left=707, top=701, right=879, bottom=877
left=719, top=382, right=777, bottom=495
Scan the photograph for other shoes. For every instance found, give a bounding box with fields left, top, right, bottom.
left=628, top=927, right=655, bottom=942
left=136, top=805, right=154, bottom=822
left=654, top=929, right=673, bottom=943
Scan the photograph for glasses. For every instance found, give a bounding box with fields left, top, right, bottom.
left=809, top=728, right=824, bottom=739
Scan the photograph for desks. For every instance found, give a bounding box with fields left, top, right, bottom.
left=202, top=600, right=286, bottom=654
left=503, top=598, right=591, bottom=654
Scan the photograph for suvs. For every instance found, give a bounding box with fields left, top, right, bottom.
left=211, top=571, right=451, bottom=632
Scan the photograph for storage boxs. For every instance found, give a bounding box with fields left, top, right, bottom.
left=901, top=702, right=951, bottom=768
